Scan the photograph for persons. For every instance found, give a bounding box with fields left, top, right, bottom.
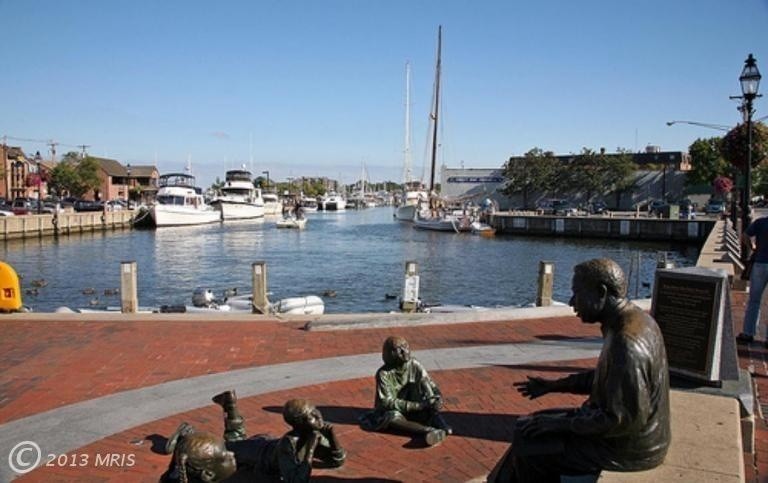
left=513, top=258, right=671, bottom=482
left=164, top=389, right=346, bottom=483
left=486, top=203, right=495, bottom=227
left=173, top=430, right=238, bottom=483
left=736, top=214, right=768, bottom=345
left=357, top=335, right=454, bottom=447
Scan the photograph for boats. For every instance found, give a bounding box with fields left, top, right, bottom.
left=54, top=295, right=326, bottom=315
left=471, top=227, right=496, bottom=237
left=461, top=219, right=497, bottom=238
left=206, top=161, right=395, bottom=231
left=148, top=154, right=222, bottom=226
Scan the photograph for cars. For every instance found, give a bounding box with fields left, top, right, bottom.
left=0, top=196, right=154, bottom=216
left=538, top=198, right=578, bottom=216
left=706, top=198, right=727, bottom=214
left=650, top=200, right=692, bottom=214
left=582, top=201, right=609, bottom=213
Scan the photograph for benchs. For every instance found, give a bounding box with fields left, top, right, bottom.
left=592, top=390, right=746, bottom=483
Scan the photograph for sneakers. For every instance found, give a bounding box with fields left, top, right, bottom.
left=735, top=333, right=754, bottom=342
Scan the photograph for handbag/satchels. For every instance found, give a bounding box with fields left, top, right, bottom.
left=740, top=259, right=754, bottom=281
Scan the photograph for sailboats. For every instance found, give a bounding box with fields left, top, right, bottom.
left=396, top=24, right=463, bottom=231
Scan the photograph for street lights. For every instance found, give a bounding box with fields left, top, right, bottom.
left=729, top=54, right=763, bottom=266
left=34, top=151, right=43, bottom=215
left=126, top=163, right=131, bottom=209
left=666, top=120, right=740, bottom=231
